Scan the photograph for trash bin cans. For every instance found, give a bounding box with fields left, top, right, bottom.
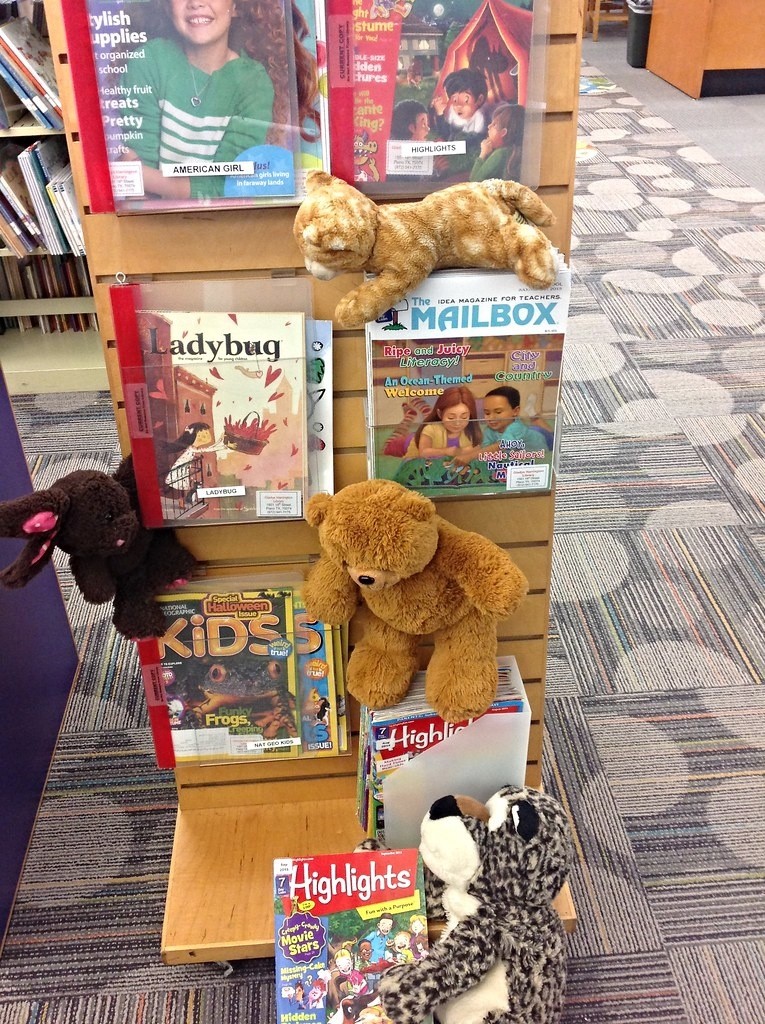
left=627, top=0, right=653, bottom=68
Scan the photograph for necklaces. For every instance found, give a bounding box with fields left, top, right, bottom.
left=189, top=65, right=210, bottom=107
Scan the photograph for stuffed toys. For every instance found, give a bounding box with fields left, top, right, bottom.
left=0, top=447, right=197, bottom=639
left=301, top=478, right=528, bottom=721
left=354, top=785, right=573, bottom=1024
left=293, top=169, right=557, bottom=327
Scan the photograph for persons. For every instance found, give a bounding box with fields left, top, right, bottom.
left=414, top=386, right=484, bottom=458
left=108, top=0, right=317, bottom=201
left=449, top=385, right=549, bottom=468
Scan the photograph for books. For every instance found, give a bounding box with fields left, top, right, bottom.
left=273, top=850, right=434, bottom=1024
left=357, top=662, right=524, bottom=840
left=0, top=0, right=99, bottom=336
left=136, top=582, right=349, bottom=767
left=63, top=0, right=572, bottom=526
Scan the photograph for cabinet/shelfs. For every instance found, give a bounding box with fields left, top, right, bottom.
left=0, top=0, right=112, bottom=394
